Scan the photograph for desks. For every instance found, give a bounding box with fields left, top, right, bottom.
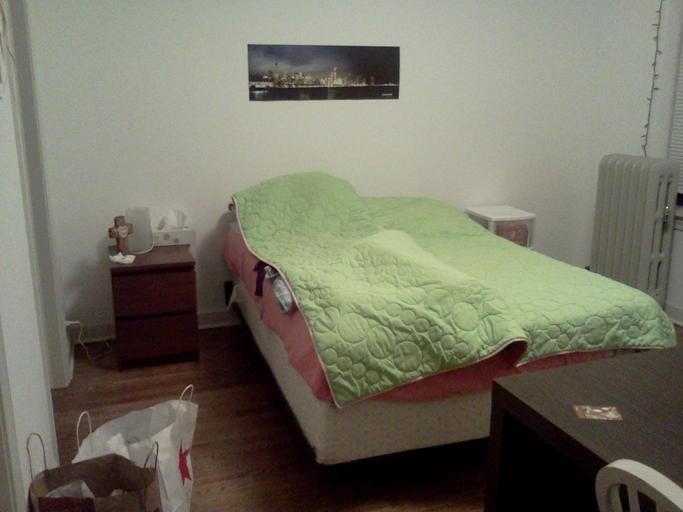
left=491, top=346, right=683, bottom=512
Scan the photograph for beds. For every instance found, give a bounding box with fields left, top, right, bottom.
left=221, top=174, right=677, bottom=465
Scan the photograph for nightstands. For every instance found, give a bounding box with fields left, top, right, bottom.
left=108, top=245, right=199, bottom=368
left=464, top=206, right=537, bottom=251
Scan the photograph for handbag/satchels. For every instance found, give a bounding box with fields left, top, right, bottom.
left=27, top=454, right=164, bottom=512
left=70, top=400, right=198, bottom=512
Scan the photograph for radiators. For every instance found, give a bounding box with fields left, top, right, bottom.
left=592, top=154, right=679, bottom=310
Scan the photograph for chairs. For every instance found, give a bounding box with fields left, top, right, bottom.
left=595, top=459, right=683, bottom=512
